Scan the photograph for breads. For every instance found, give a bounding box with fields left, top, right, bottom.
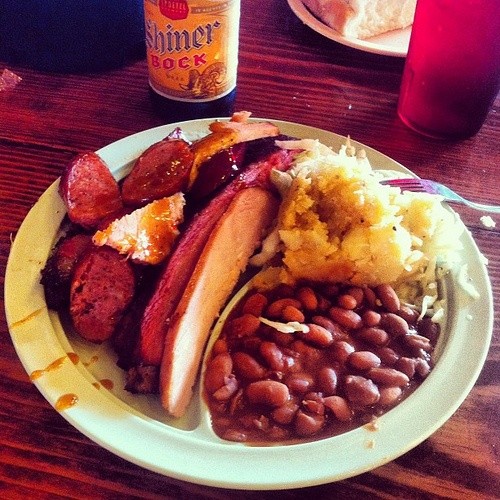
left=304, top=0, right=416, bottom=40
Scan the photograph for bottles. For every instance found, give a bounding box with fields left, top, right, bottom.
left=399, top=0, right=499, bottom=146
left=145, top=1, right=241, bottom=118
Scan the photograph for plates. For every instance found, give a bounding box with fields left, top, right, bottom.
left=2, top=117, right=495, bottom=490
left=288, top=1, right=420, bottom=60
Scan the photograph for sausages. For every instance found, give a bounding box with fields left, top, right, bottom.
left=123, top=135, right=193, bottom=206
left=69, top=244, right=133, bottom=342
left=44, top=231, right=93, bottom=308
left=60, top=148, right=123, bottom=231
